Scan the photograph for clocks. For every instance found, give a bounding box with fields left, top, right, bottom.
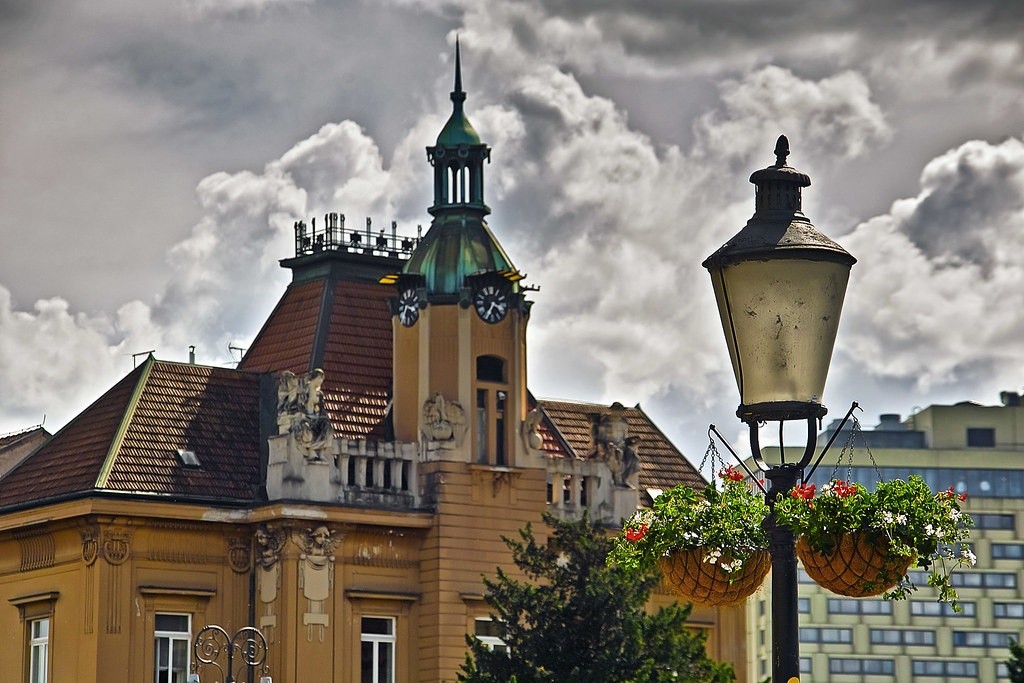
left=396, top=289, right=419, bottom=330
left=474, top=285, right=507, bottom=323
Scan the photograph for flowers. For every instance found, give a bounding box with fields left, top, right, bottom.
left=775, top=482, right=978, bottom=609
left=604, top=463, right=768, bottom=586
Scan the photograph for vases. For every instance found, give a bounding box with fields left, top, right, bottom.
left=656, top=546, right=773, bottom=604
left=798, top=530, right=914, bottom=602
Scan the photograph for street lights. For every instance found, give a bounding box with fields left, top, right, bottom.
left=703, top=132, right=857, bottom=683
